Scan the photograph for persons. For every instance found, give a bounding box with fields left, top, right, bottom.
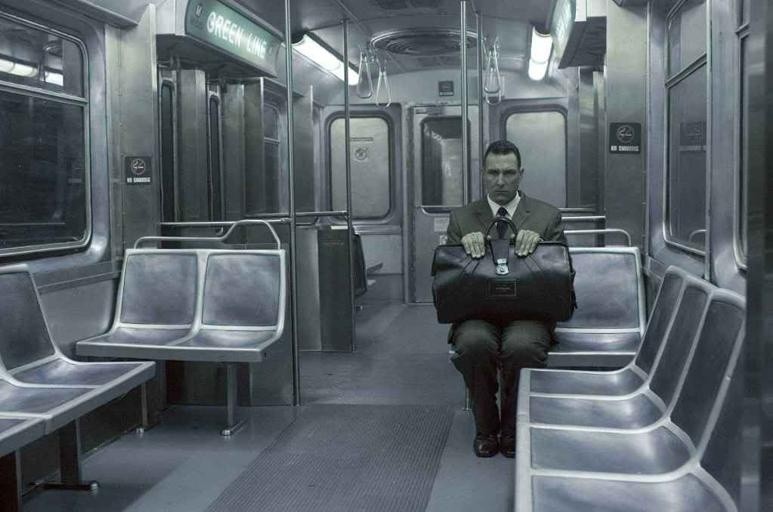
left=444, top=138, right=565, bottom=457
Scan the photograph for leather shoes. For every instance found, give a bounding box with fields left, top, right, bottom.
left=474, top=430, right=515, bottom=458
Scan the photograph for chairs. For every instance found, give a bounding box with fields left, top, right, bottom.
left=512, top=263, right=746, bottom=511
left=296, top=212, right=383, bottom=311
left=462, top=244, right=647, bottom=413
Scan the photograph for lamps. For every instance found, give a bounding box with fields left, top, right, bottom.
left=525, top=25, right=554, bottom=82
left=281, top=29, right=366, bottom=88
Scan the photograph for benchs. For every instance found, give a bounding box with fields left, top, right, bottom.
left=0, top=262, right=156, bottom=492
left=69, top=220, right=291, bottom=436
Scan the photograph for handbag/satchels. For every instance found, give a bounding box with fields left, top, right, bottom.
left=431, top=240, right=575, bottom=324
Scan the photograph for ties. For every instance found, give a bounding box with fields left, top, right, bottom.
left=497, top=208, right=508, bottom=239
